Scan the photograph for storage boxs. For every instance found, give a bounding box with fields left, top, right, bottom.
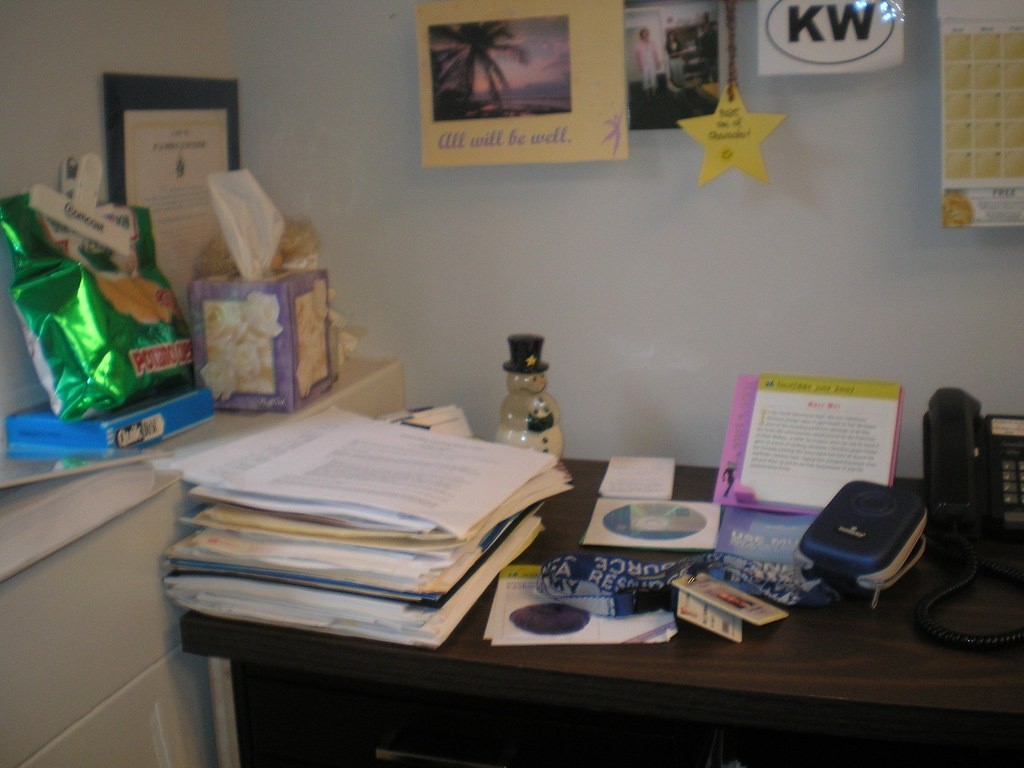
left=188, top=267, right=341, bottom=411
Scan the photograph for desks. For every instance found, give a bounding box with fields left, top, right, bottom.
left=182, top=454, right=1024, bottom=768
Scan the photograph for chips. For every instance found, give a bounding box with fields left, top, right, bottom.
left=96, top=276, right=169, bottom=324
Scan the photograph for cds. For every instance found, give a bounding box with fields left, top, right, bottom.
left=582, top=496, right=723, bottom=552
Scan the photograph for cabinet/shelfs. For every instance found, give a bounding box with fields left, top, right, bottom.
left=0, top=360, right=406, bottom=768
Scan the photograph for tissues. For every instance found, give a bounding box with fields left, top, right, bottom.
left=188, top=167, right=339, bottom=413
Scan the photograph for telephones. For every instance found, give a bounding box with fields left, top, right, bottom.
left=919, top=386, right=1023, bottom=547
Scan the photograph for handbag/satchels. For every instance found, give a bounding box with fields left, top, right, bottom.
left=798, top=479, right=929, bottom=610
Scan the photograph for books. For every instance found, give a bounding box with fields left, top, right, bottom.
left=160, top=402, right=575, bottom=651
